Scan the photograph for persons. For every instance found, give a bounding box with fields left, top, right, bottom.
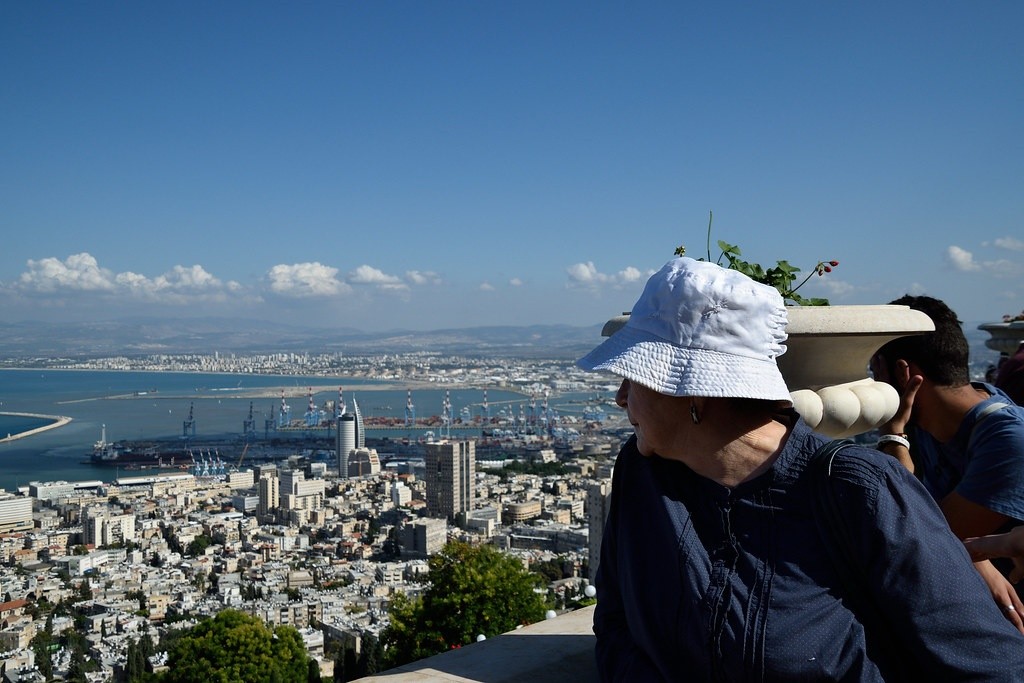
left=572, top=256, right=1024, bottom=683
left=994, top=340, right=1024, bottom=407
left=870, top=296, right=1024, bottom=534
left=962, top=527, right=1024, bottom=584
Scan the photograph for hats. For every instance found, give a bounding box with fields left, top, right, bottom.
left=574, top=257, right=793, bottom=407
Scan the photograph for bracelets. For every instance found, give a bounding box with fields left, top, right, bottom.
left=878, top=433, right=910, bottom=450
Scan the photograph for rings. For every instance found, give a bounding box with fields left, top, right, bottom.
left=1002, top=605, right=1014, bottom=613
